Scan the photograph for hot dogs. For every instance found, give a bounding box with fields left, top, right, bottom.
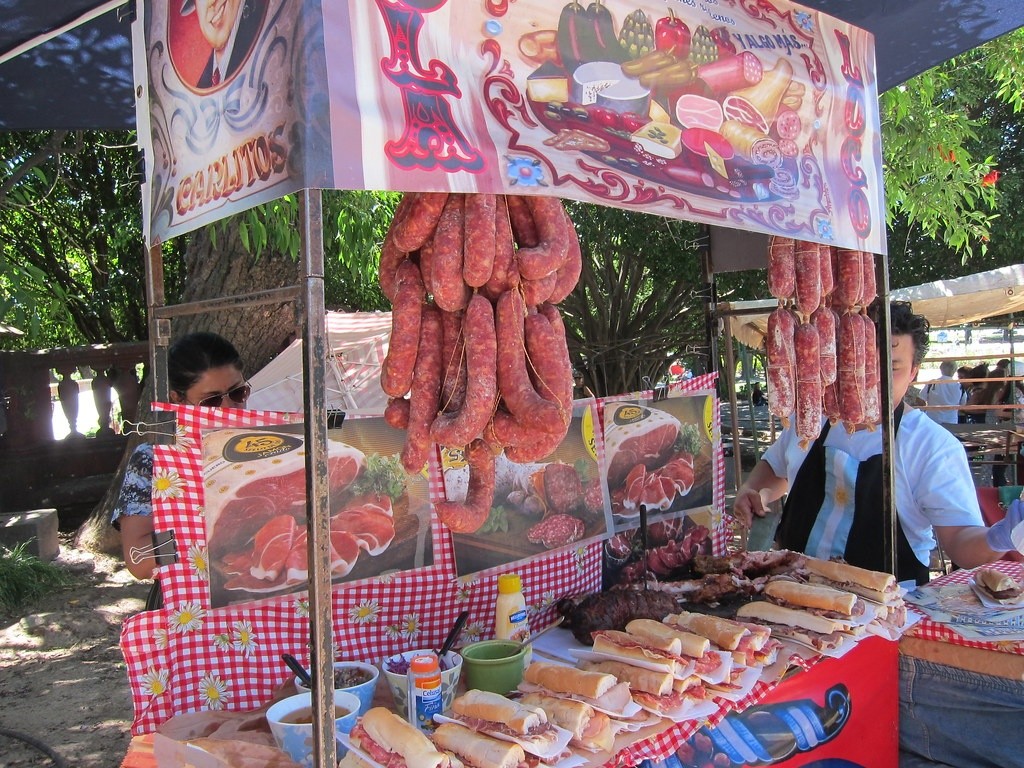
left=347, top=559, right=908, bottom=768
left=973, top=567, right=1024, bottom=605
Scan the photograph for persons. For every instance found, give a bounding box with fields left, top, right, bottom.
left=734, top=302, right=1024, bottom=592
left=917, top=359, right=1024, bottom=427
left=119, top=331, right=251, bottom=611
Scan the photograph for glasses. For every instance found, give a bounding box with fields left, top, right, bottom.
left=201, top=380, right=252, bottom=408
left=958, top=375, right=965, bottom=379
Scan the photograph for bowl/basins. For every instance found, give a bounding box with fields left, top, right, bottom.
left=381, top=649, right=463, bottom=717
left=265, top=691, right=361, bottom=768
left=460, top=639, right=527, bottom=695
left=294, top=661, right=380, bottom=717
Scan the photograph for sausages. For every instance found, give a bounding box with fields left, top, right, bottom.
left=378, top=192, right=583, bottom=532
left=765, top=235, right=880, bottom=450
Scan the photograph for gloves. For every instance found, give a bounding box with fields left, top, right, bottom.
left=986, top=499, right=1024, bottom=553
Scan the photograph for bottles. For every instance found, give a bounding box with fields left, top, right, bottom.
left=406, top=655, right=443, bottom=735
left=495, top=575, right=533, bottom=670
